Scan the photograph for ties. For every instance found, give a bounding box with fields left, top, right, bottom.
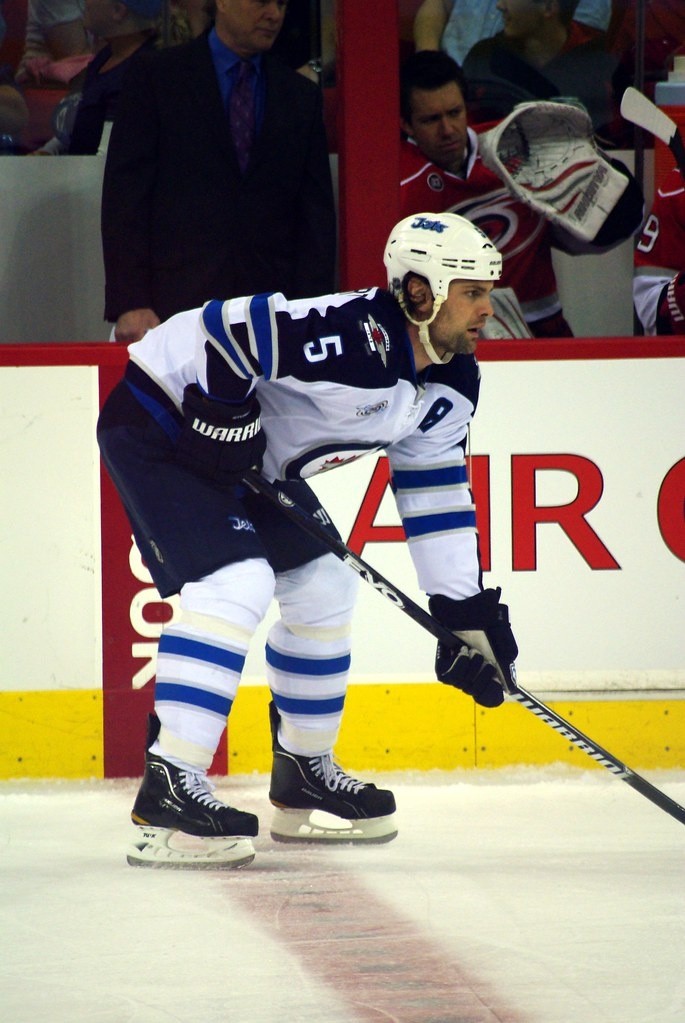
left=228, top=61, right=255, bottom=171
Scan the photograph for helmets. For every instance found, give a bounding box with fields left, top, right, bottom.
left=383, top=212, right=503, bottom=305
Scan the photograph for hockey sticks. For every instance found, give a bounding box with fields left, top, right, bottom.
left=240, top=468, right=685, bottom=828
left=618, top=84, right=684, bottom=180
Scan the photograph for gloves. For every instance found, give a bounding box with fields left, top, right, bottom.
left=181, top=384, right=266, bottom=486
left=428, top=587, right=519, bottom=708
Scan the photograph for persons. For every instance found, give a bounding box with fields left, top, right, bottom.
left=633, top=166, right=685, bottom=338
left=271, top=0, right=322, bottom=84
left=101, top=0, right=336, bottom=344
left=0, top=0, right=216, bottom=156
left=605, top=0, right=685, bottom=76
left=96, top=212, right=519, bottom=870
left=399, top=51, right=646, bottom=342
left=412, top=0, right=611, bottom=68
left=463, top=0, right=637, bottom=149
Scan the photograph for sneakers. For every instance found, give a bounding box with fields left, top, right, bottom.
left=269, top=699, right=398, bottom=844
left=126, top=712, right=258, bottom=870
